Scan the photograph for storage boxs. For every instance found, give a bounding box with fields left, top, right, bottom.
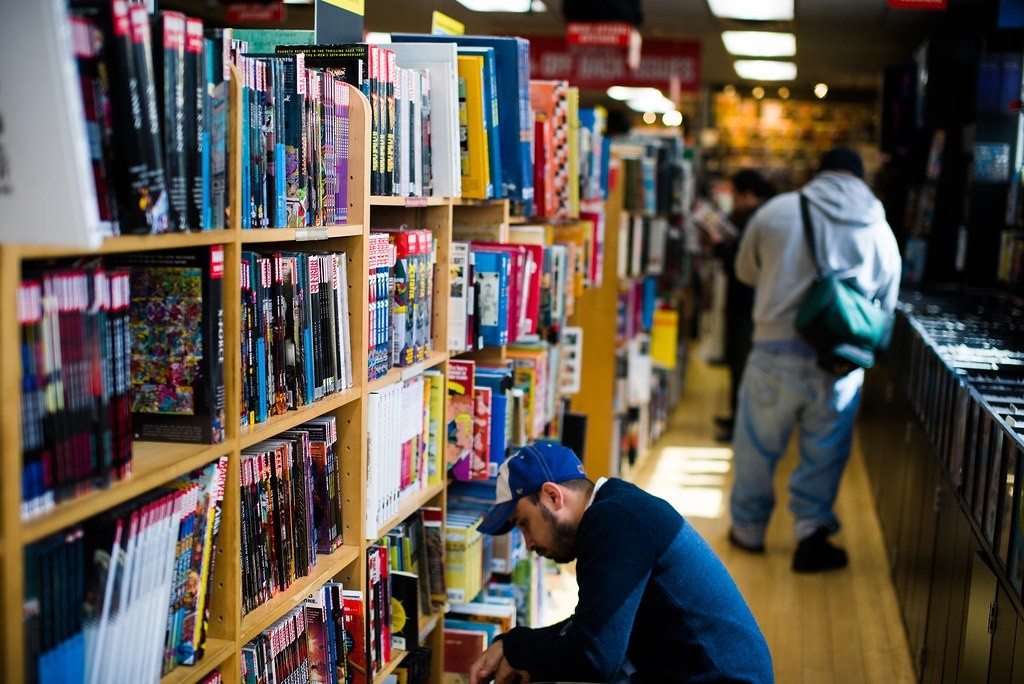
left=887, top=285, right=1023, bottom=604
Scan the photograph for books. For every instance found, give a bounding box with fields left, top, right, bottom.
left=0, top=2, right=699, bottom=684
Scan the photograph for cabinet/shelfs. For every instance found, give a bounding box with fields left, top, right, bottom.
left=858, top=355, right=1024, bottom=684
left=2, top=77, right=711, bottom=683
left=712, top=80, right=883, bottom=175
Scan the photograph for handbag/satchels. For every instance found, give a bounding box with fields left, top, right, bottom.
left=795, top=276, right=897, bottom=373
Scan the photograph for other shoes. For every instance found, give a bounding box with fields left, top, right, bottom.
left=715, top=413, right=737, bottom=444
left=729, top=525, right=847, bottom=573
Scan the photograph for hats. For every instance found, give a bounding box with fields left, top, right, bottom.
left=477, top=440, right=585, bottom=536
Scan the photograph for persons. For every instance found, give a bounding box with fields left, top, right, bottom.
left=469, top=440, right=775, bottom=684
left=706, top=167, right=775, bottom=438
left=727, top=146, right=903, bottom=572
left=700, top=170, right=735, bottom=367
